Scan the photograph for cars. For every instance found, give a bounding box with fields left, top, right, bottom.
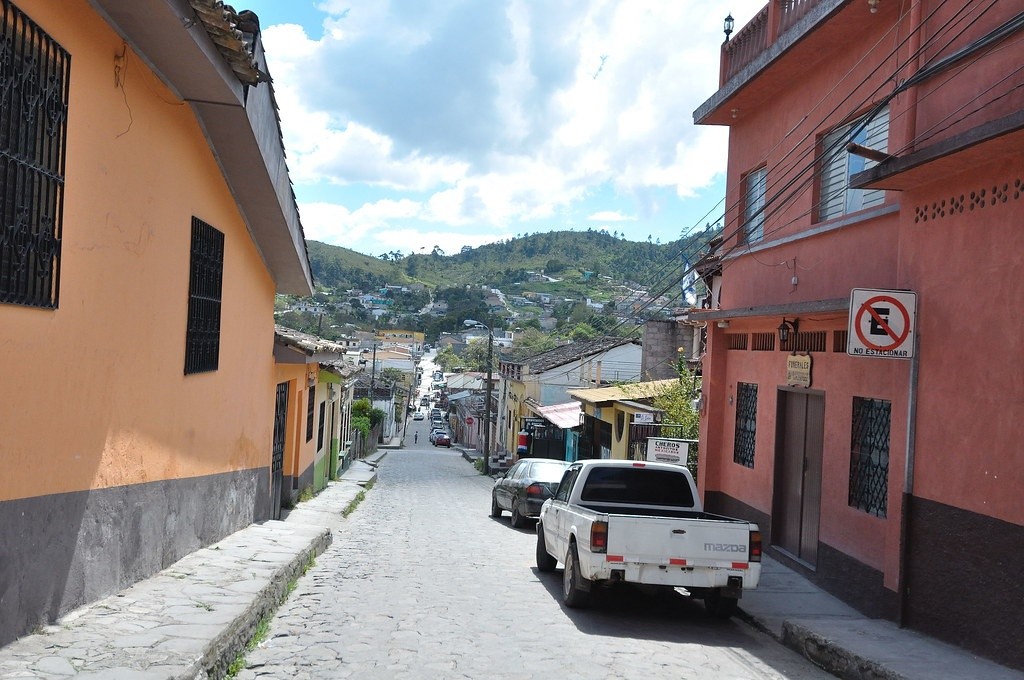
left=421, top=370, right=450, bottom=448
left=414, top=413, right=424, bottom=420
left=491, top=457, right=574, bottom=527
left=424, top=345, right=430, bottom=353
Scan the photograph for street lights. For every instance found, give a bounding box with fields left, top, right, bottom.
left=464, top=320, right=490, bottom=474
left=370, top=347, right=383, bottom=409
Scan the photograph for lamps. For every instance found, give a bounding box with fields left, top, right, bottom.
left=723, top=11, right=735, bottom=43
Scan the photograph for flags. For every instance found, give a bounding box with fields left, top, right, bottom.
left=682, top=263, right=697, bottom=305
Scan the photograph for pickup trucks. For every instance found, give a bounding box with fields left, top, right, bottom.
left=535, top=460, right=763, bottom=625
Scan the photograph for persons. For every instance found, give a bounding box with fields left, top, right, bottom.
left=414, top=431, right=418, bottom=444
left=425, top=399, right=428, bottom=409
left=431, top=382, right=432, bottom=388
left=427, top=411, right=430, bottom=420
